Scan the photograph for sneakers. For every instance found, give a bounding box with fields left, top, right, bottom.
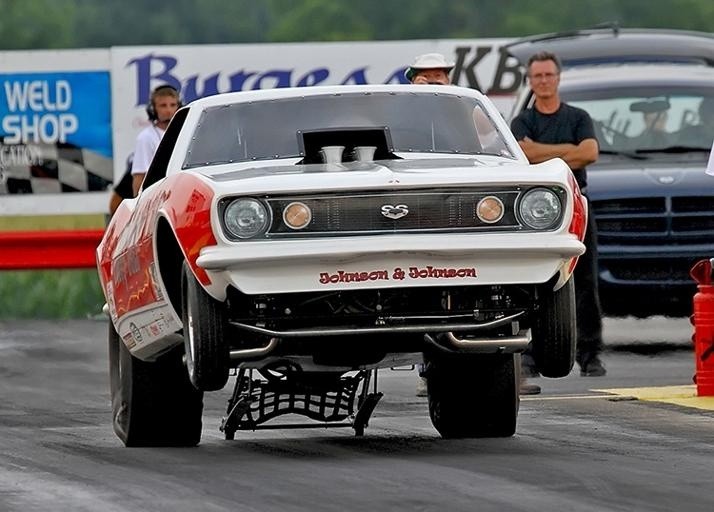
left=521, top=365, right=538, bottom=379
left=520, top=379, right=540, bottom=395
left=581, top=359, right=606, bottom=377
left=416, top=378, right=429, bottom=395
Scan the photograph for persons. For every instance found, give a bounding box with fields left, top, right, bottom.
left=404, top=53, right=540, bottom=398
left=109, top=162, right=133, bottom=218
left=508, top=53, right=609, bottom=380
left=129, top=86, right=182, bottom=200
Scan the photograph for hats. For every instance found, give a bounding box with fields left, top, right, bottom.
left=405, top=53, right=455, bottom=81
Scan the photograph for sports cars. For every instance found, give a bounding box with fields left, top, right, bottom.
left=89, top=79, right=591, bottom=448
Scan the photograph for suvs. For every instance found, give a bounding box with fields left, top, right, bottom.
left=493, top=20, right=714, bottom=317
